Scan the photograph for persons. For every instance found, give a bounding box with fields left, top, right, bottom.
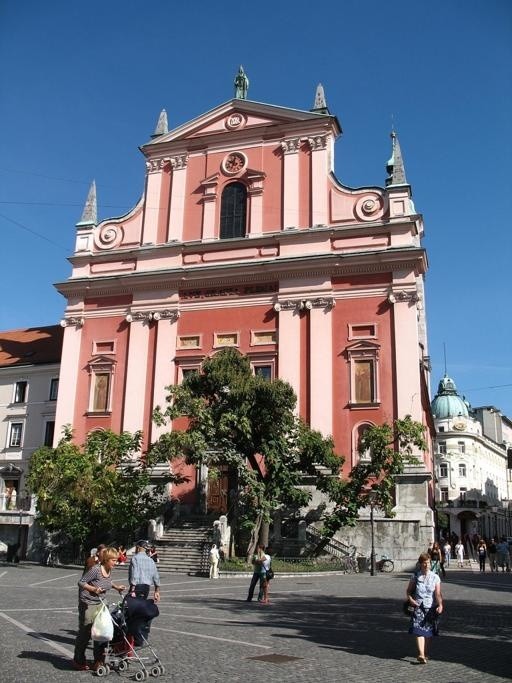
left=7, top=487, right=18, bottom=510
left=257, top=546, right=273, bottom=603
left=427, top=530, right=512, bottom=578
left=208, top=544, right=219, bottom=579
left=406, top=552, right=443, bottom=664
left=72, top=539, right=162, bottom=671
left=245, top=544, right=266, bottom=602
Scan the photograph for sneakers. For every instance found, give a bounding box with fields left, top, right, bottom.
left=72, top=659, right=90, bottom=670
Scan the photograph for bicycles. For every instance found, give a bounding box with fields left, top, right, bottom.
left=343, top=555, right=360, bottom=574
left=366, top=553, right=395, bottom=572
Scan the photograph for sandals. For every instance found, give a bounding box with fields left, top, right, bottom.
left=418, top=655, right=426, bottom=664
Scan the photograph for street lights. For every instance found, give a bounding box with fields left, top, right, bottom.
left=474, top=496, right=509, bottom=572
left=368, top=483, right=380, bottom=576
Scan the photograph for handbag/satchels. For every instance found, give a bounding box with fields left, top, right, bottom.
left=404, top=598, right=414, bottom=615
left=266, top=568, right=274, bottom=579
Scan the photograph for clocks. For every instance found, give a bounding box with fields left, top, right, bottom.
left=221, top=151, right=248, bottom=175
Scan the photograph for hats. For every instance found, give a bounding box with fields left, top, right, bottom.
left=135, top=539, right=151, bottom=550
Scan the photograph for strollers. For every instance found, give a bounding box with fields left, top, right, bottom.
left=89, top=586, right=165, bottom=681
